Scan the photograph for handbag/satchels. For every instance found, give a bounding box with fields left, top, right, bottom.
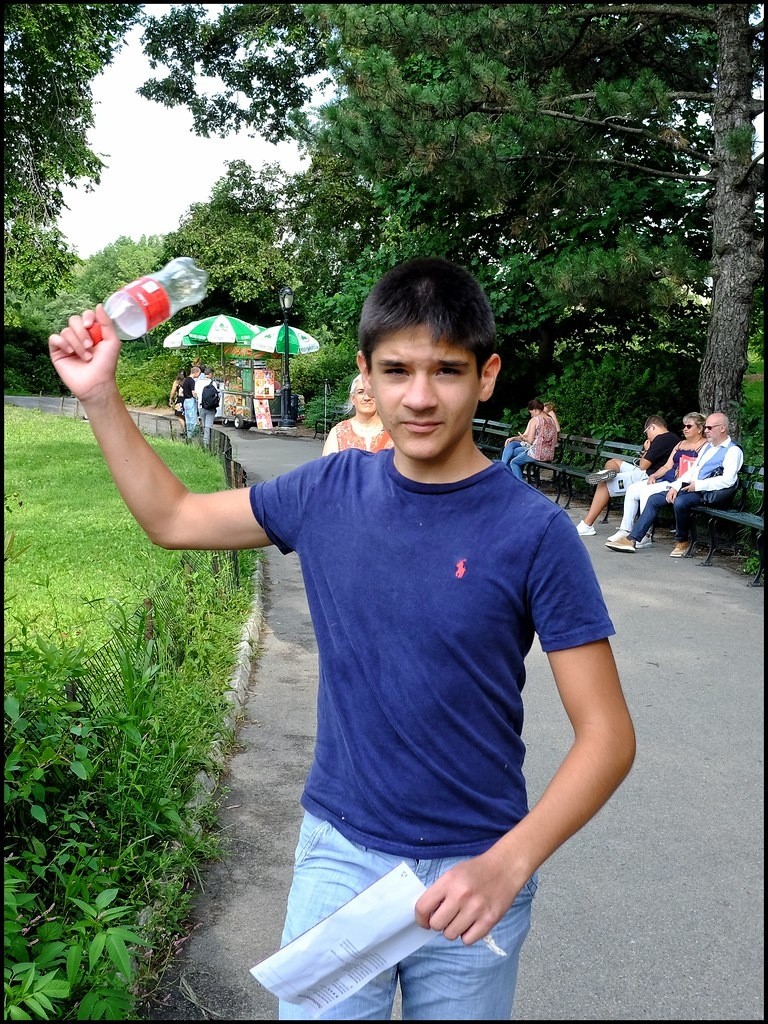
left=701, top=467, right=741, bottom=505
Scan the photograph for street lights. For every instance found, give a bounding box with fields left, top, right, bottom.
left=278, top=286, right=297, bottom=428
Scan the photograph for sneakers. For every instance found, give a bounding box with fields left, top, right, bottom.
left=576, top=520, right=597, bottom=536
left=604, top=538, right=637, bottom=553
left=670, top=542, right=691, bottom=558
left=585, top=470, right=617, bottom=485
left=636, top=535, right=653, bottom=548
left=606, top=530, right=626, bottom=542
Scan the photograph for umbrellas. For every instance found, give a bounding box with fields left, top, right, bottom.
left=163, top=313, right=319, bottom=389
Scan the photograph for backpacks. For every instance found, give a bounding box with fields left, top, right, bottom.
left=202, top=381, right=220, bottom=410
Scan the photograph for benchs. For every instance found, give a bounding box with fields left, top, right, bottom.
left=313, top=401, right=355, bottom=439
left=472, top=418, right=486, bottom=444
left=521, top=433, right=764, bottom=588
left=475, top=420, right=513, bottom=460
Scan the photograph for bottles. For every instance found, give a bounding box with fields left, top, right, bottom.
left=225, top=376, right=242, bottom=391
left=86, top=255, right=211, bottom=343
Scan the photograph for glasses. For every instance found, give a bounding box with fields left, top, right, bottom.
left=682, top=423, right=699, bottom=429
left=703, top=425, right=721, bottom=431
left=643, top=425, right=653, bottom=435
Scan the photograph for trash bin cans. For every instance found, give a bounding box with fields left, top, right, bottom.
left=291, top=394, right=299, bottom=421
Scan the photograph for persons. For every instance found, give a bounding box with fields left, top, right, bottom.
left=49, top=258, right=637, bottom=1021
left=322, top=374, right=394, bottom=455
left=169, top=358, right=221, bottom=449
left=501, top=399, right=561, bottom=480
left=576, top=412, right=744, bottom=557
